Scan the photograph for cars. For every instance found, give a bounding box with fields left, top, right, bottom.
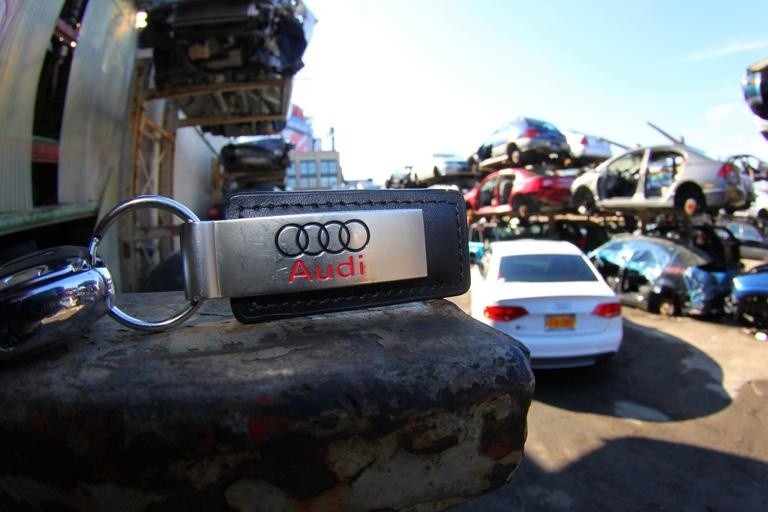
left=138, top=0, right=318, bottom=220
